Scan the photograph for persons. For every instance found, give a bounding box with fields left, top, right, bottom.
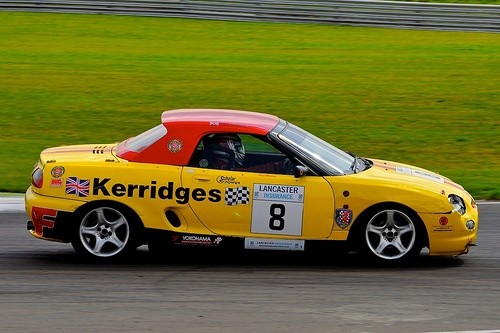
left=201, top=131, right=292, bottom=172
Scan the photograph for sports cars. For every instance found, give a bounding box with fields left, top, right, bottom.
left=23, top=106, right=479, bottom=266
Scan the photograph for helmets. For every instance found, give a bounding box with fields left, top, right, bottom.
left=210, top=133, right=245, bottom=165
